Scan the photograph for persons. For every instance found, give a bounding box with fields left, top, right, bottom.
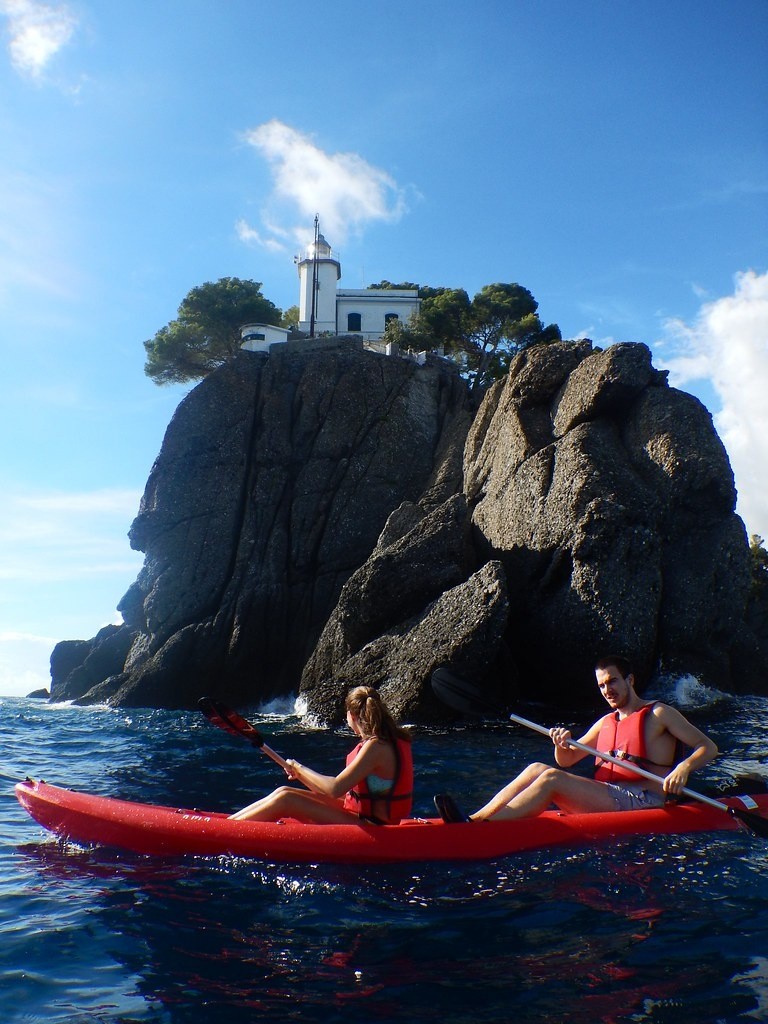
left=226, top=685, right=411, bottom=826
left=433, top=655, right=719, bottom=825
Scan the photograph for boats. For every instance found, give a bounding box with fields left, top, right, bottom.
left=15, top=777, right=768, bottom=862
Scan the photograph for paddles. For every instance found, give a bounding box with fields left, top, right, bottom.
left=199, top=696, right=315, bottom=791
left=510, top=711, right=768, bottom=837
